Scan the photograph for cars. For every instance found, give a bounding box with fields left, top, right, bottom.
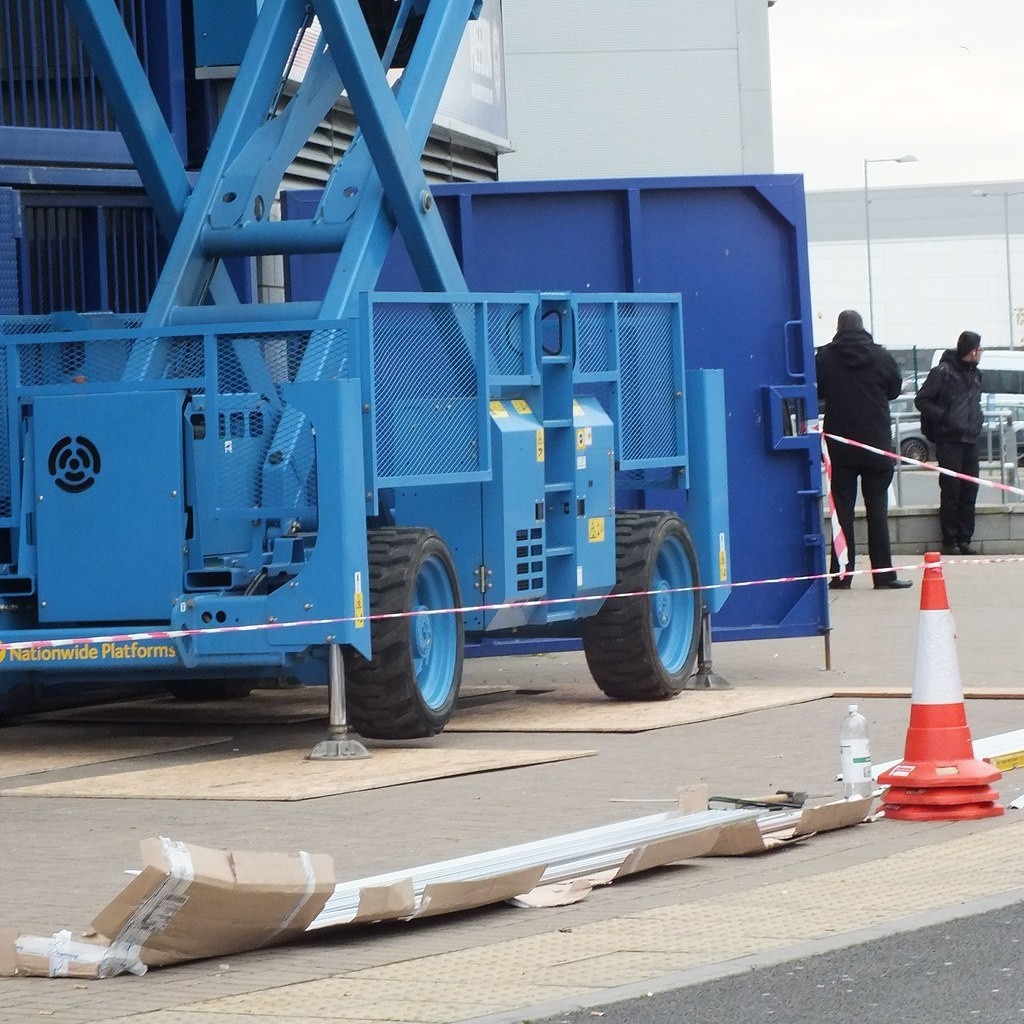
left=882, top=354, right=1024, bottom=466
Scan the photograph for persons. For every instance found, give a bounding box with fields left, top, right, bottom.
left=816, top=310, right=913, bottom=589
left=914, top=331, right=982, bottom=558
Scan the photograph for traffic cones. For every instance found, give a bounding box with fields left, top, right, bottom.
left=878, top=551, right=1005, bottom=819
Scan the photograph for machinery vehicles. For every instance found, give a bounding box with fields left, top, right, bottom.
left=0, top=0, right=736, bottom=750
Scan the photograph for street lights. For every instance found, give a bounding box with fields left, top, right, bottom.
left=971, top=187, right=1015, bottom=353
left=865, top=156, right=918, bottom=344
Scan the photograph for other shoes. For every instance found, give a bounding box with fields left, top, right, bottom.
left=829, top=576, right=852, bottom=590
left=958, top=540, right=978, bottom=555
left=944, top=543, right=961, bottom=555
left=874, top=579, right=913, bottom=589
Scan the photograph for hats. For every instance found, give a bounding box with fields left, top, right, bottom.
left=957, top=331, right=981, bottom=354
left=837, top=310, right=863, bottom=333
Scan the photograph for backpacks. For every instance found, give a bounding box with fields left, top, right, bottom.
left=920, top=362, right=949, bottom=443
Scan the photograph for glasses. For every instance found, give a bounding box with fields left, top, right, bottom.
left=976, top=347, right=985, bottom=352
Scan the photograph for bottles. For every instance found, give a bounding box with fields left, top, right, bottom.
left=838, top=704, right=872, bottom=802
